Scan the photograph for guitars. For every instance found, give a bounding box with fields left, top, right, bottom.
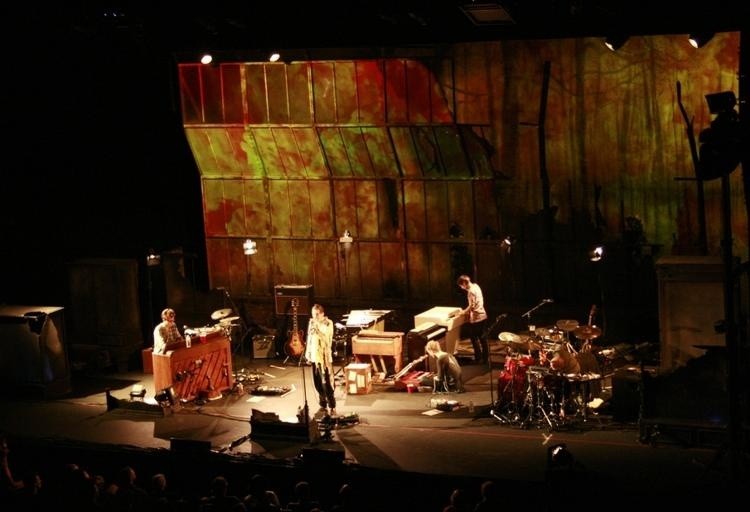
left=283, top=297, right=307, bottom=361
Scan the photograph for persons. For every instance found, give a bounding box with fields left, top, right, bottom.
left=0, top=436, right=506, bottom=512
left=456, top=275, right=489, bottom=363
left=154, top=308, right=182, bottom=351
left=425, top=341, right=465, bottom=394
left=304, top=305, right=338, bottom=418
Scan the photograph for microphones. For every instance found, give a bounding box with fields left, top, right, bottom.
left=542, top=299, right=552, bottom=302
left=311, top=321, right=318, bottom=334
left=496, top=313, right=507, bottom=323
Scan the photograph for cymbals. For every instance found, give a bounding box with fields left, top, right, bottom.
left=534, top=325, right=558, bottom=341
left=518, top=339, right=544, bottom=352
left=573, top=324, right=603, bottom=341
left=497, top=331, right=528, bottom=345
left=220, top=316, right=242, bottom=323
left=556, top=319, right=581, bottom=332
left=221, top=323, right=241, bottom=326
left=211, top=307, right=233, bottom=320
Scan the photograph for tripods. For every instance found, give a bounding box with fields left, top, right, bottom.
left=473, top=324, right=509, bottom=424
left=298, top=335, right=309, bottom=416
left=217, top=287, right=265, bottom=376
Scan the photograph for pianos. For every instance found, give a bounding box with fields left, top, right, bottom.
left=392, top=353, right=436, bottom=394
left=406, top=321, right=448, bottom=374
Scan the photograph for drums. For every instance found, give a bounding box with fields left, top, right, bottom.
left=496, top=352, right=534, bottom=416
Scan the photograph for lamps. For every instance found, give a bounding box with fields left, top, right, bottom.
left=503, top=235, right=514, bottom=247
left=242, top=238, right=258, bottom=256
left=338, top=227, right=354, bottom=244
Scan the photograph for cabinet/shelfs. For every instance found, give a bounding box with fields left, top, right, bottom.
left=273, top=284, right=312, bottom=316
left=150, top=332, right=234, bottom=404
left=654, top=256, right=726, bottom=374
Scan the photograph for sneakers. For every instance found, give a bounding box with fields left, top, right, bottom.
left=331, top=409, right=337, bottom=416
left=315, top=409, right=327, bottom=417
